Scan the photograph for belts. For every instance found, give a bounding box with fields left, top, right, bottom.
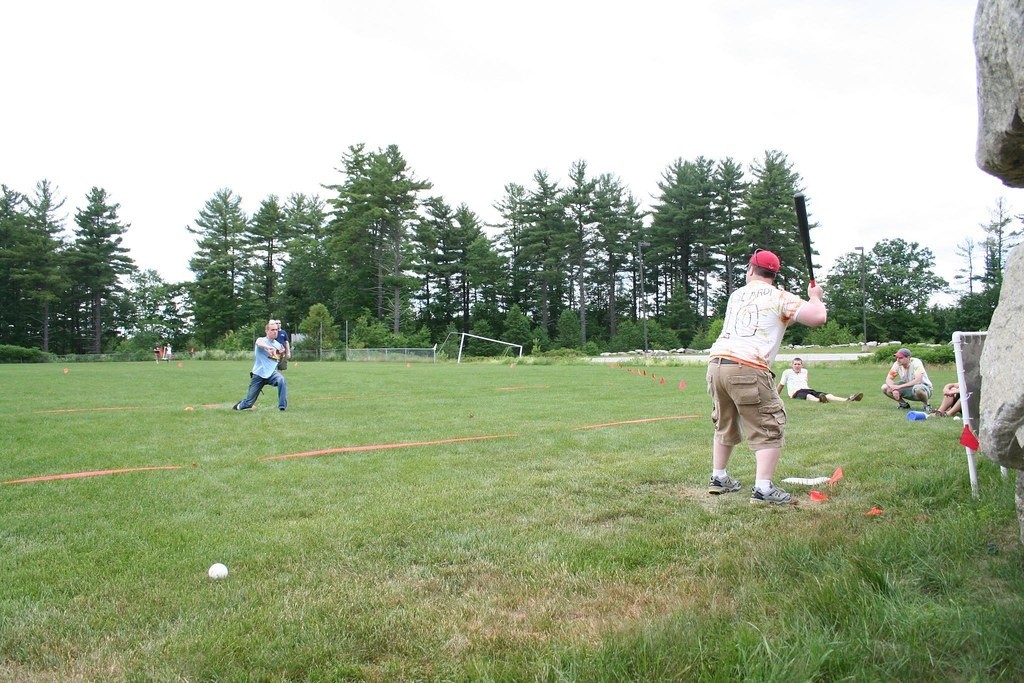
left=710, top=358, right=739, bottom=364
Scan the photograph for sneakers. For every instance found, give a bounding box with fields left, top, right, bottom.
left=749, top=482, right=792, bottom=505
left=709, top=475, right=741, bottom=493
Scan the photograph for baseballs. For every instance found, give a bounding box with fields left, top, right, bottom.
left=208, top=563, right=228, bottom=579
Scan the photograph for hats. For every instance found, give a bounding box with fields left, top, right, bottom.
left=749, top=250, right=780, bottom=272
left=893, top=348, right=911, bottom=357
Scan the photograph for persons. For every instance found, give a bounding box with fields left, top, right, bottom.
left=777, top=358, right=863, bottom=402
left=706, top=248, right=828, bottom=503
left=231, top=320, right=289, bottom=411
left=274, top=319, right=291, bottom=372
left=928, top=383, right=962, bottom=417
left=153, top=341, right=161, bottom=365
left=166, top=343, right=172, bottom=362
left=880, top=348, right=933, bottom=411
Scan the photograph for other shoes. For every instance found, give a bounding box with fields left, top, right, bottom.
left=233, top=401, right=242, bottom=410
left=280, top=408, right=285, bottom=411
left=930, top=409, right=948, bottom=418
left=897, top=403, right=910, bottom=409
left=819, top=394, right=829, bottom=403
left=924, top=403, right=932, bottom=412
left=847, top=392, right=864, bottom=401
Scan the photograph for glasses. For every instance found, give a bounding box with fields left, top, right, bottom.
left=754, top=248, right=764, bottom=267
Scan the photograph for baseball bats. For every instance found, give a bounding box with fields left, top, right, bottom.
left=795, top=193, right=816, bottom=288
